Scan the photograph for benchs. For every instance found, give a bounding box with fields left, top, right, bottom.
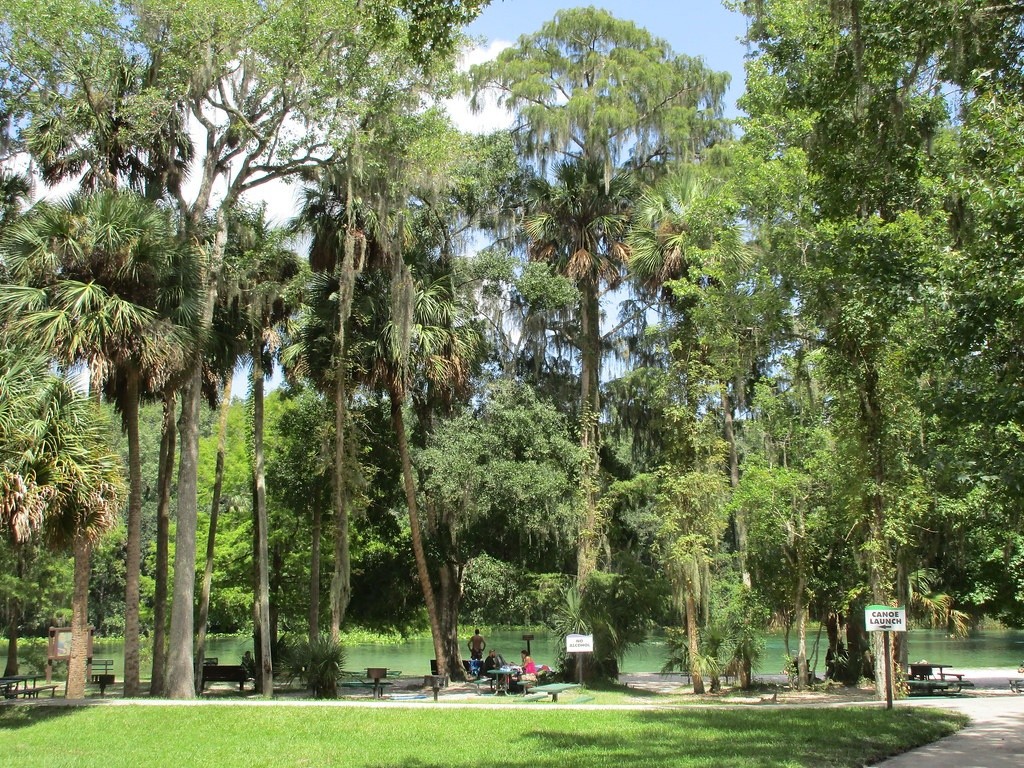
left=573, top=696, right=595, bottom=704
left=517, top=678, right=541, bottom=697
left=793, top=661, right=815, bottom=684
left=66, top=659, right=114, bottom=674
left=473, top=678, right=493, bottom=696
left=1007, top=678, right=1024, bottom=693
left=681, top=664, right=732, bottom=685
left=300, top=682, right=387, bottom=697
left=202, top=665, right=280, bottom=691
left=430, top=659, right=484, bottom=675
left=6, top=685, right=59, bottom=699
left=513, top=695, right=548, bottom=703
left=905, top=674, right=975, bottom=696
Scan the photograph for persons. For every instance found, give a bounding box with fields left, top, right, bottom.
left=481, top=650, right=538, bottom=681
left=240, top=651, right=255, bottom=674
left=467, top=629, right=486, bottom=660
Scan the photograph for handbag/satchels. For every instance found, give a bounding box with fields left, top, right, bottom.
left=521, top=672, right=539, bottom=682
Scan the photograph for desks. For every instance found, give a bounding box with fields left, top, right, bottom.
left=527, top=684, right=582, bottom=702
left=908, top=664, right=953, bottom=693
left=487, top=666, right=524, bottom=697
left=0, top=674, right=45, bottom=699
left=339, top=671, right=402, bottom=694
